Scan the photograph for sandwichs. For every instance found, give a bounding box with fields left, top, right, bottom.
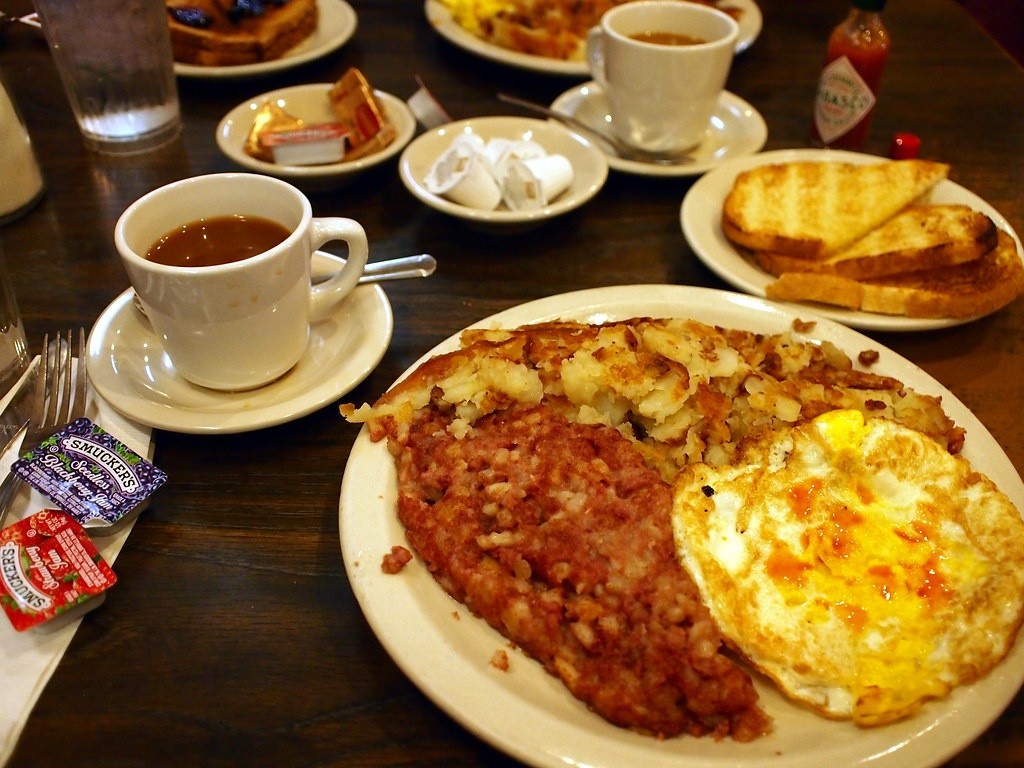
left=165, top=0, right=316, bottom=67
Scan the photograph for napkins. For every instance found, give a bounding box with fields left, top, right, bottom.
left=0, top=353, right=155, bottom=768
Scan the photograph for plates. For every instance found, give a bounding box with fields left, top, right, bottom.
left=174, top=0, right=358, bottom=76
left=399, top=117, right=609, bottom=221
left=427, top=0, right=762, bottom=73
left=681, top=150, right=1024, bottom=331
left=216, top=84, right=415, bottom=176
left=340, top=284, right=1024, bottom=767
left=547, top=80, right=768, bottom=176
left=86, top=250, right=393, bottom=433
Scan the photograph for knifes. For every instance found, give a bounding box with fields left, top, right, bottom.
left=0, top=339, right=68, bottom=459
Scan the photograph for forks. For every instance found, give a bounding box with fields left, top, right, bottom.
left=0, top=328, right=87, bottom=528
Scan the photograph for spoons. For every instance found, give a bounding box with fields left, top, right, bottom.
left=501, top=96, right=691, bottom=166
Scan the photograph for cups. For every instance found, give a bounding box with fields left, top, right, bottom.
left=587, top=1, right=738, bottom=151
left=36, top=0, right=181, bottom=156
left=115, top=173, right=368, bottom=393
left=0, top=78, right=46, bottom=226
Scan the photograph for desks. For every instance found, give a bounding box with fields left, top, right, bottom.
left=0, top=0, right=1024, bottom=768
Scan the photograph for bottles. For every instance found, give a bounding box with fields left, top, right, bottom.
left=808, top=1, right=891, bottom=153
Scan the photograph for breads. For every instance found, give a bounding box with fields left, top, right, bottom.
left=720, top=158, right=1024, bottom=319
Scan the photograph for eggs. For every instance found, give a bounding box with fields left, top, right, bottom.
left=670, top=409, right=1024, bottom=726
